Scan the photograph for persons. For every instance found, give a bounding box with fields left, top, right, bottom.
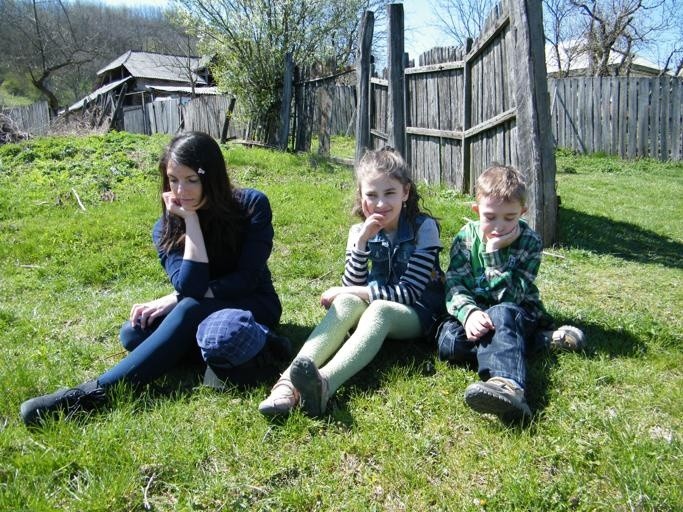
left=17, top=129, right=304, bottom=435
left=255, top=145, right=446, bottom=422
left=433, top=161, right=588, bottom=427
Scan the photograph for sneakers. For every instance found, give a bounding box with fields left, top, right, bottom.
left=20, top=380, right=109, bottom=434
left=464, top=377, right=531, bottom=429
left=538, top=325, right=586, bottom=351
left=258, top=355, right=330, bottom=420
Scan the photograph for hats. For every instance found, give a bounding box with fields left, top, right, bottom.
left=196, top=309, right=291, bottom=389
